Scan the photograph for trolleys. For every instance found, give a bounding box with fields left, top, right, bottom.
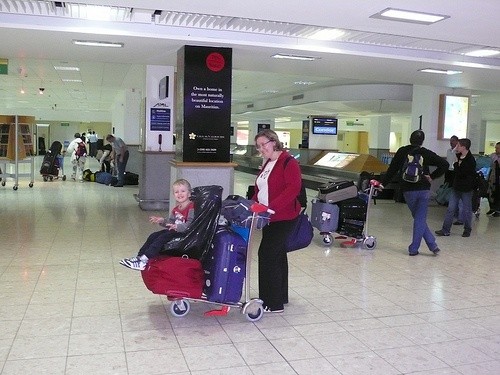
left=42, top=151, right=66, bottom=181
left=166, top=211, right=265, bottom=322
left=320, top=180, right=384, bottom=251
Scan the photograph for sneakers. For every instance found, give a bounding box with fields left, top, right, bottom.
left=119, top=257, right=146, bottom=271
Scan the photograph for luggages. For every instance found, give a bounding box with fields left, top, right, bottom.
left=310, top=180, right=367, bottom=238
left=203, top=227, right=246, bottom=303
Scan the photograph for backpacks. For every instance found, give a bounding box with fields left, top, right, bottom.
left=400, top=147, right=426, bottom=184
left=74, top=140, right=87, bottom=157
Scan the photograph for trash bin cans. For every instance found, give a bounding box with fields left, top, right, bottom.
left=64, top=141, right=70, bottom=151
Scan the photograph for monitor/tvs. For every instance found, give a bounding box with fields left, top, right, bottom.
left=313, top=118, right=338, bottom=135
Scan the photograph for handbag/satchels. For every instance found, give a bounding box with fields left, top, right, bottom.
left=140, top=253, right=206, bottom=299
left=285, top=212, right=314, bottom=253
left=83, top=169, right=117, bottom=186
left=435, top=182, right=452, bottom=205
left=221, top=195, right=270, bottom=229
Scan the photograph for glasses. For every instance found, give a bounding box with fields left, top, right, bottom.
left=256, top=140, right=272, bottom=150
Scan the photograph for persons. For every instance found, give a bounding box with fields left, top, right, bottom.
left=251, top=129, right=302, bottom=313
left=487, top=142, right=500, bottom=217
left=435, top=136, right=476, bottom=237
left=69, top=131, right=98, bottom=183
left=382, top=130, right=449, bottom=256
left=120, top=179, right=195, bottom=270
left=98, top=135, right=129, bottom=187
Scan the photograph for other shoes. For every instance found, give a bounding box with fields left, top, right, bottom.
left=435, top=230, right=450, bottom=236
left=492, top=212, right=500, bottom=217
left=409, top=252, right=419, bottom=256
left=433, top=248, right=440, bottom=254
left=453, top=222, right=463, bottom=225
left=262, top=305, right=284, bottom=312
left=462, top=231, right=470, bottom=237
left=486, top=210, right=494, bottom=215
left=73, top=178, right=75, bottom=181
left=114, top=185, right=123, bottom=187
